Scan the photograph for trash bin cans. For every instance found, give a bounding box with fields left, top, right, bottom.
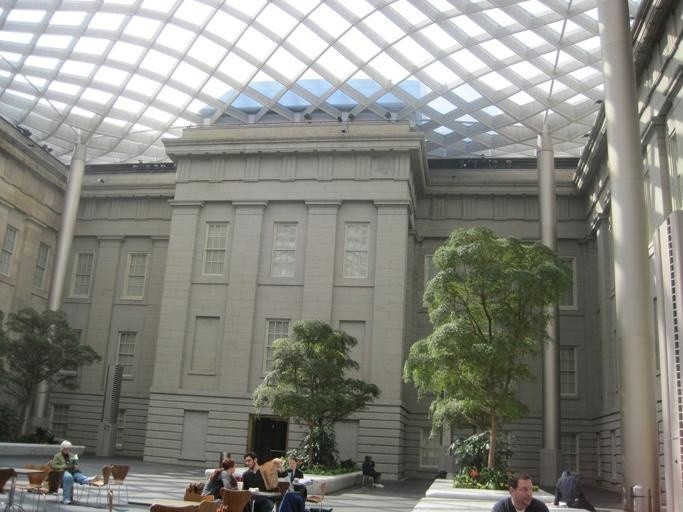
left=630, top=484, right=651, bottom=512
left=219, top=451, right=231, bottom=468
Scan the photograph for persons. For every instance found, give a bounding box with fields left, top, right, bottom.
left=240, top=451, right=275, bottom=512
left=283, top=454, right=303, bottom=483
left=202, top=457, right=237, bottom=498
left=51, top=439, right=98, bottom=504
left=489, top=471, right=550, bottom=511
left=360, top=455, right=384, bottom=488
left=552, top=469, right=597, bottom=512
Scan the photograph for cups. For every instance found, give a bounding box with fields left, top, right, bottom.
left=237, top=482, right=244, bottom=491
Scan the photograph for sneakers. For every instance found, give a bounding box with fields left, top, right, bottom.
left=372, top=482, right=384, bottom=488
left=87, top=475, right=101, bottom=484
left=64, top=500, right=73, bottom=504
